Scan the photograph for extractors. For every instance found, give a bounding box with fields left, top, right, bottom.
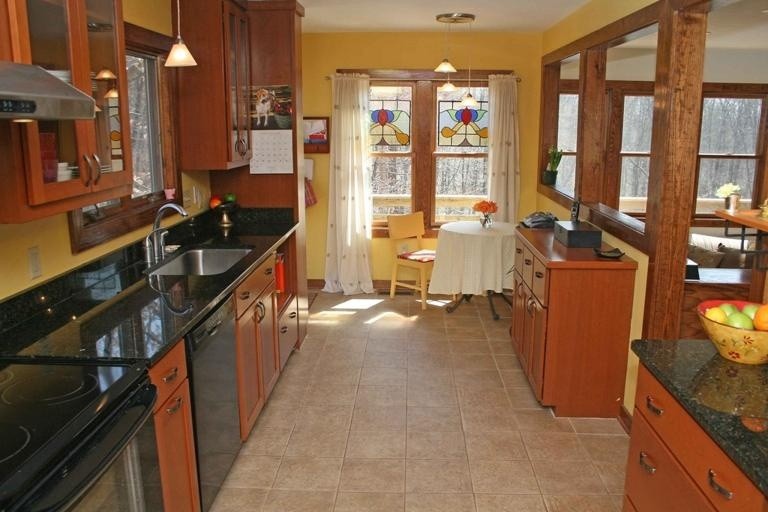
left=0, top=62, right=96, bottom=121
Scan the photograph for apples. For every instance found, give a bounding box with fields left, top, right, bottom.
left=720, top=302, right=740, bottom=317
left=726, top=311, right=754, bottom=331
left=741, top=303, right=760, bottom=321
left=208, top=195, right=223, bottom=209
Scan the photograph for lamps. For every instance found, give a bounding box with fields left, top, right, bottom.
left=434, top=12, right=478, bottom=107
left=88, top=22, right=119, bottom=99
left=164, top=0, right=197, bottom=67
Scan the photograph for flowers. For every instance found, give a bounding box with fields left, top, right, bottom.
left=716, top=183, right=739, bottom=198
left=472, top=200, right=498, bottom=226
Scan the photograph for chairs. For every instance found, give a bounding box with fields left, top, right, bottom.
left=387, top=211, right=456, bottom=311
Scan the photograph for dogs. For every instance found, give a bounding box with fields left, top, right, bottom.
left=255, top=88, right=276, bottom=127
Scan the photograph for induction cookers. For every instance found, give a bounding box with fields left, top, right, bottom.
left=0, top=365, right=131, bottom=480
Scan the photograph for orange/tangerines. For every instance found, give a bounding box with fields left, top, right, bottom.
left=751, top=303, right=768, bottom=332
left=473, top=199, right=497, bottom=213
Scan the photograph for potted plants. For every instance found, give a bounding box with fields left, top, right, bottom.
left=541, top=144, right=563, bottom=185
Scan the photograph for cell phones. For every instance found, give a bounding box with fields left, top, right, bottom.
left=569, top=199, right=579, bottom=221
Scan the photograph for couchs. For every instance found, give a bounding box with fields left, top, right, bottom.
left=691, top=234, right=748, bottom=252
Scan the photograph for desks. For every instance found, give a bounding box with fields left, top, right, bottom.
left=427, top=221, right=515, bottom=320
left=715, top=209, right=768, bottom=271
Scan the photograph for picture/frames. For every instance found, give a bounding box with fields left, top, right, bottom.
left=303, top=116, right=330, bottom=154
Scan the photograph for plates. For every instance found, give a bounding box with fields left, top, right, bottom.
left=68, top=162, right=113, bottom=176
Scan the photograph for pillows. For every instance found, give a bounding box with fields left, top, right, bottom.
left=687, top=243, right=726, bottom=268
left=718, top=243, right=768, bottom=269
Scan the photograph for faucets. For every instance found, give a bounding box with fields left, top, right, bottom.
left=153, top=202, right=188, bottom=247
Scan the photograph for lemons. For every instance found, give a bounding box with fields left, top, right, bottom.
left=704, top=306, right=726, bottom=324
left=223, top=192, right=236, bottom=203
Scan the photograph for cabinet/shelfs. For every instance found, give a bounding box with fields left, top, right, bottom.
left=509, top=226, right=638, bottom=418
left=148, top=339, right=201, bottom=512
left=622, top=339, right=768, bottom=512
left=277, top=293, right=297, bottom=372
left=233, top=254, right=280, bottom=442
left=0, top=0, right=133, bottom=224
left=173, top=0, right=251, bottom=172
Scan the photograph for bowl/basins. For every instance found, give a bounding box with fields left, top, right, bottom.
left=695, top=300, right=768, bottom=366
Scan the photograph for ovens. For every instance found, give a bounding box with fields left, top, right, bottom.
left=0, top=375, right=164, bottom=511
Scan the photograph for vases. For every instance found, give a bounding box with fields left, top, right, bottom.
left=482, top=213, right=492, bottom=228
left=726, top=194, right=738, bottom=210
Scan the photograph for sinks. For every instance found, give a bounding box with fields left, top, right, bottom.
left=155, top=250, right=247, bottom=274
